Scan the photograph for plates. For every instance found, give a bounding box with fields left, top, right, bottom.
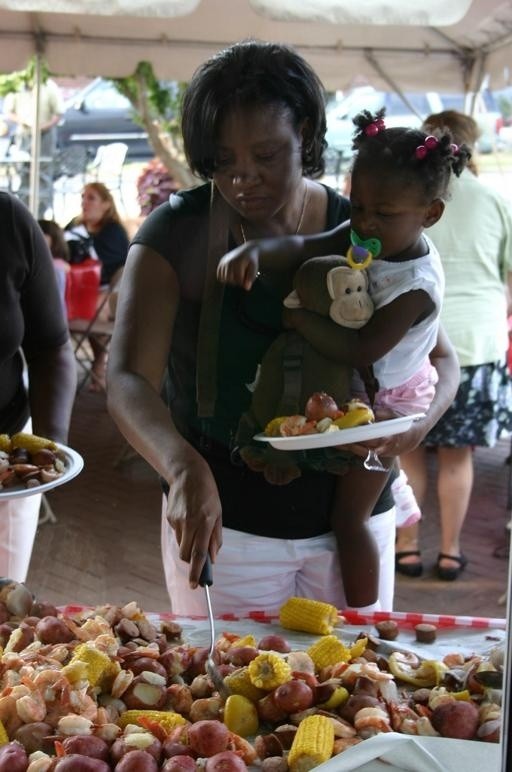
left=249, top=412, right=429, bottom=454
left=0, top=436, right=90, bottom=502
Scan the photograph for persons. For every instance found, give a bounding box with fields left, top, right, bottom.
left=33, top=215, right=72, bottom=314
left=63, top=184, right=128, bottom=384
left=100, top=38, right=465, bottom=622
left=1, top=191, right=83, bottom=594
left=213, top=108, right=472, bottom=616
left=385, top=104, right=510, bottom=579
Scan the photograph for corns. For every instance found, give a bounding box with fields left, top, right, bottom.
left=249, top=596, right=369, bottom=772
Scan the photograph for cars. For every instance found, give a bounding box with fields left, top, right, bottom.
left=14, top=72, right=180, bottom=162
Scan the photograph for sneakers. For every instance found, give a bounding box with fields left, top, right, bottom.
left=389, top=483, right=423, bottom=528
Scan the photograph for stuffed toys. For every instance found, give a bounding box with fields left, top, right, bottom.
left=231, top=242, right=378, bottom=492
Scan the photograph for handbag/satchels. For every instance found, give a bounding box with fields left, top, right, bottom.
left=67, top=229, right=94, bottom=264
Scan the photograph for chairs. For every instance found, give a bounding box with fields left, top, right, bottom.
left=68, top=266, right=121, bottom=397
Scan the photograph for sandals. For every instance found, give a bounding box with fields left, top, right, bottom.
left=395, top=550, right=423, bottom=577
left=436, top=550, right=467, bottom=581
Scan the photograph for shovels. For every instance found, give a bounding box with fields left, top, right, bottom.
left=199, top=550, right=234, bottom=701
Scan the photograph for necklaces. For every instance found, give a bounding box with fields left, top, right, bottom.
left=238, top=185, right=308, bottom=258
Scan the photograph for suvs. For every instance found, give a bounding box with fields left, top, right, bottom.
left=325, top=72, right=508, bottom=175
